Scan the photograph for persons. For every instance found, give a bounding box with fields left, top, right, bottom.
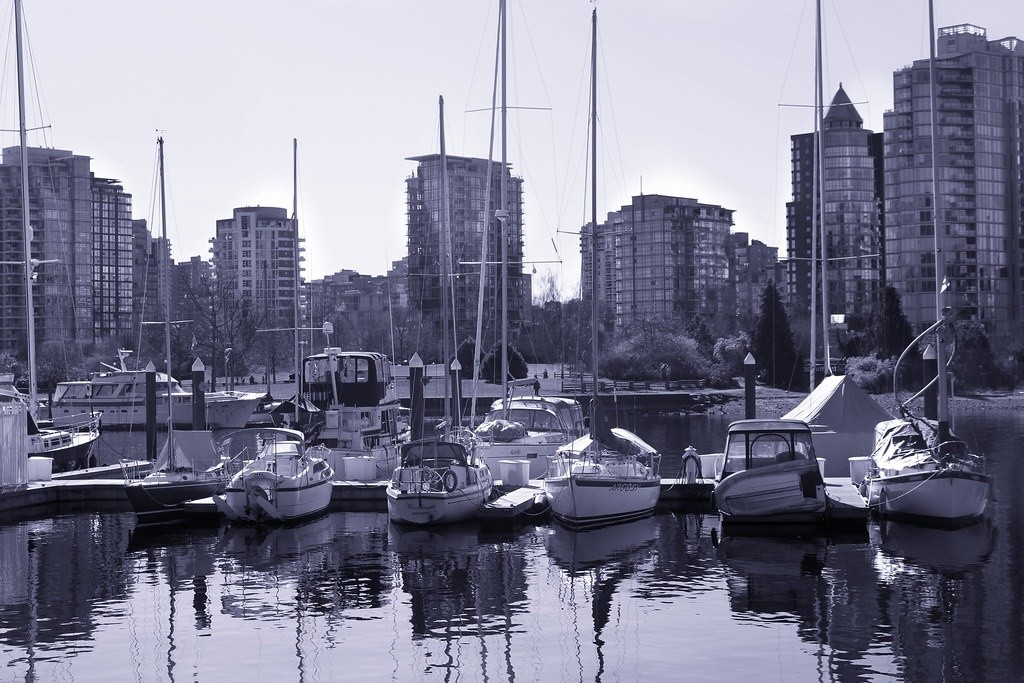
left=250, top=375, right=255, bottom=384
left=543, top=369, right=547, bottom=378
left=534, top=380, right=540, bottom=396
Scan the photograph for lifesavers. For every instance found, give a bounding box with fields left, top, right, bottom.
left=443, top=471, right=458, bottom=492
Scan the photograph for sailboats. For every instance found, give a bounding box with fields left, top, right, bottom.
left=214, top=135, right=337, bottom=523
left=0, top=0, right=102, bottom=469
left=870, top=1, right=991, bottom=526
left=386, top=93, right=493, bottom=531
left=120, top=133, right=248, bottom=526
left=545, top=6, right=663, bottom=531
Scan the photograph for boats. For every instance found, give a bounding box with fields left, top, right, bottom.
left=714, top=416, right=827, bottom=521
left=251, top=398, right=282, bottom=425
left=273, top=338, right=413, bottom=479
left=54, top=346, right=268, bottom=429
left=474, top=386, right=585, bottom=482
